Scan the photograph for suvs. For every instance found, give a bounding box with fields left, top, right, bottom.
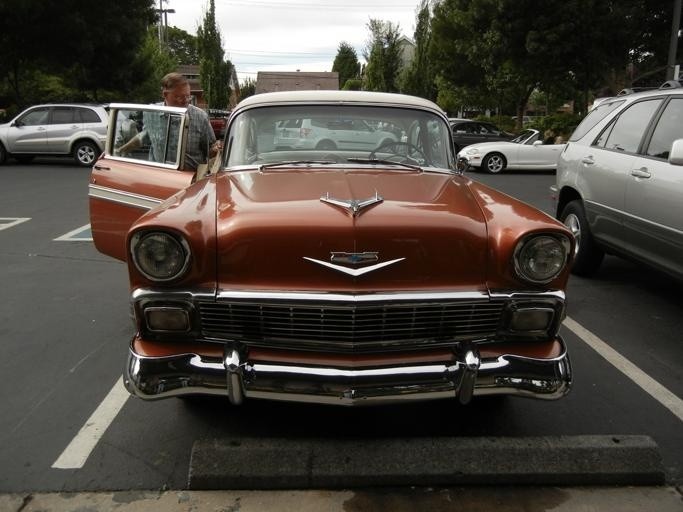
left=0, top=105, right=138, bottom=167
left=554, top=91, right=683, bottom=281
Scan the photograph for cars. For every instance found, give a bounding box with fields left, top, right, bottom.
left=274, top=116, right=399, bottom=152
left=450, top=120, right=517, bottom=153
left=88, top=91, right=576, bottom=406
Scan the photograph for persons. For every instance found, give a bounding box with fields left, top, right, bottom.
left=544, top=128, right=559, bottom=144
left=97, top=72, right=223, bottom=172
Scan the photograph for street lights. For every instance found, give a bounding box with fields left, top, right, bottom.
left=156, top=9, right=175, bottom=48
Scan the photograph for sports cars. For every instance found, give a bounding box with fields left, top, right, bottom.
left=457, top=128, right=567, bottom=174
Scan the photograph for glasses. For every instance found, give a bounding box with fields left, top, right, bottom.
left=172, top=94, right=193, bottom=102
